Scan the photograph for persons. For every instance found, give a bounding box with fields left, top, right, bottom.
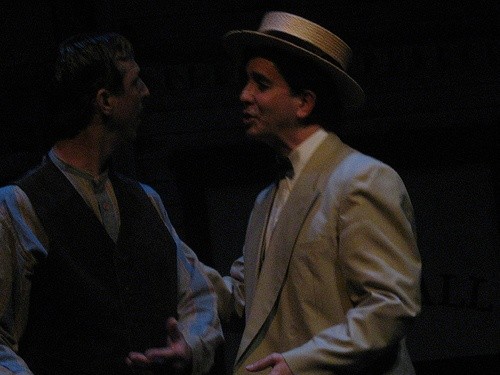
left=0, top=34, right=226, bottom=374
left=180, top=12, right=423, bottom=375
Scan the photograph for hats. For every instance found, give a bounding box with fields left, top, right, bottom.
left=224, top=11, right=366, bottom=106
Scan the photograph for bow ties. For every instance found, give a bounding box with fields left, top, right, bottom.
left=272, top=157, right=293, bottom=179
